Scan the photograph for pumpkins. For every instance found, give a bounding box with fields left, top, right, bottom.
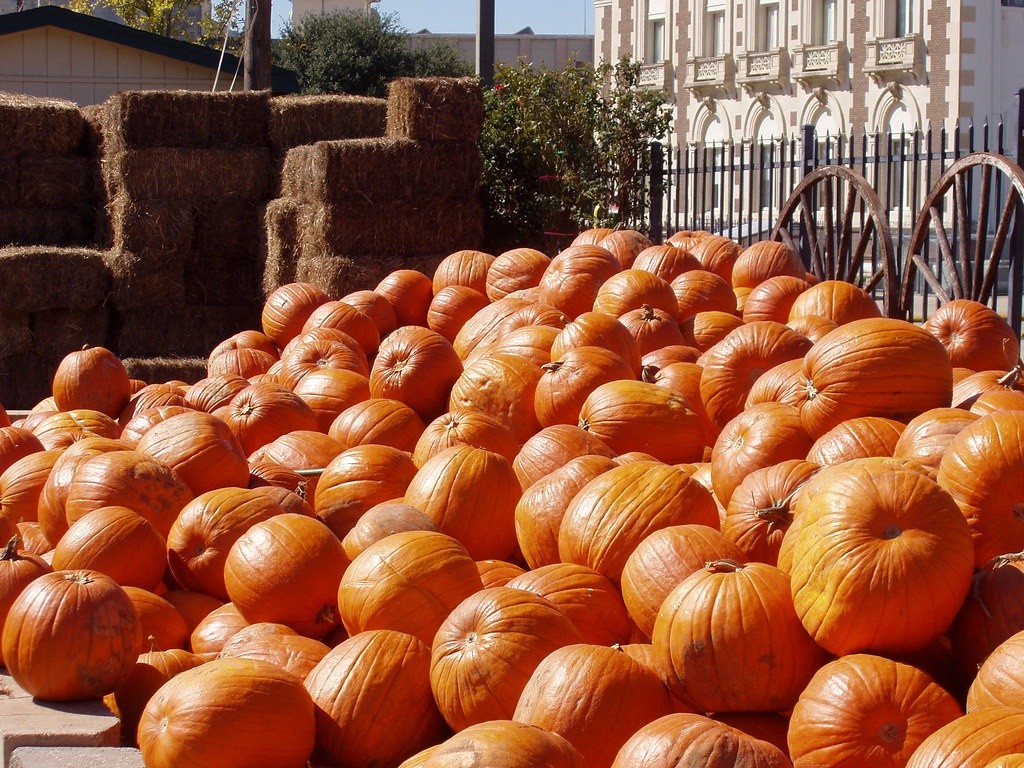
left=0, top=228, right=1024, bottom=768
